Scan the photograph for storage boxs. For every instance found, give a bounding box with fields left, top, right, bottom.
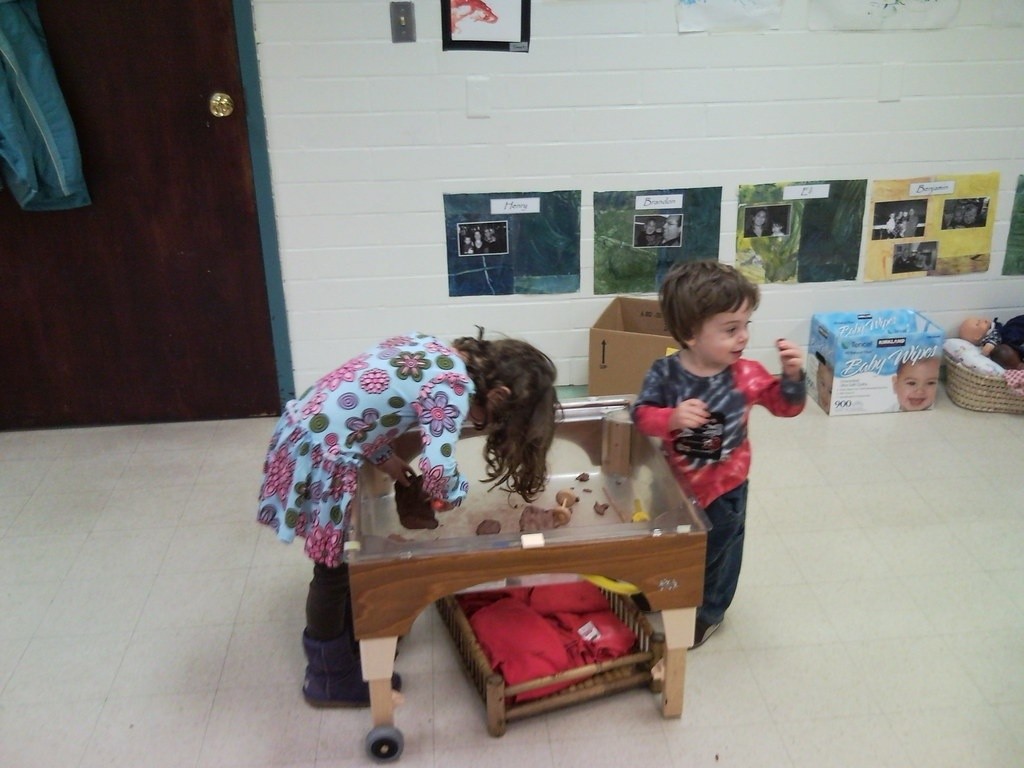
left=804, top=307, right=945, bottom=417
left=438, top=577, right=667, bottom=738
left=587, top=297, right=682, bottom=396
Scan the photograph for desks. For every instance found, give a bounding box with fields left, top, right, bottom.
left=343, top=393, right=713, bottom=761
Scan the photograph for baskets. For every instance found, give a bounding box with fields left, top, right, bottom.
left=944, top=355, right=1024, bottom=415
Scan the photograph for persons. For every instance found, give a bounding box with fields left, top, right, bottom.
left=639, top=220, right=663, bottom=247
left=769, top=219, right=786, bottom=236
left=895, top=247, right=929, bottom=271
left=892, top=356, right=941, bottom=412
left=460, top=224, right=502, bottom=255
left=945, top=202, right=980, bottom=229
left=631, top=263, right=806, bottom=651
left=257, top=328, right=564, bottom=709
left=659, top=216, right=681, bottom=247
left=885, top=208, right=919, bottom=239
left=816, top=362, right=834, bottom=415
left=960, top=314, right=1024, bottom=357
left=744, top=209, right=771, bottom=238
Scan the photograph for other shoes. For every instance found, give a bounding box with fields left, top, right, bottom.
left=688, top=610, right=729, bottom=649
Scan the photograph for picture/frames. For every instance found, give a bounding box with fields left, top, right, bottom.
left=441, top=0, right=530, bottom=53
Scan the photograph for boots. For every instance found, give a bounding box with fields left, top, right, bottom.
left=303, top=626, right=402, bottom=707
